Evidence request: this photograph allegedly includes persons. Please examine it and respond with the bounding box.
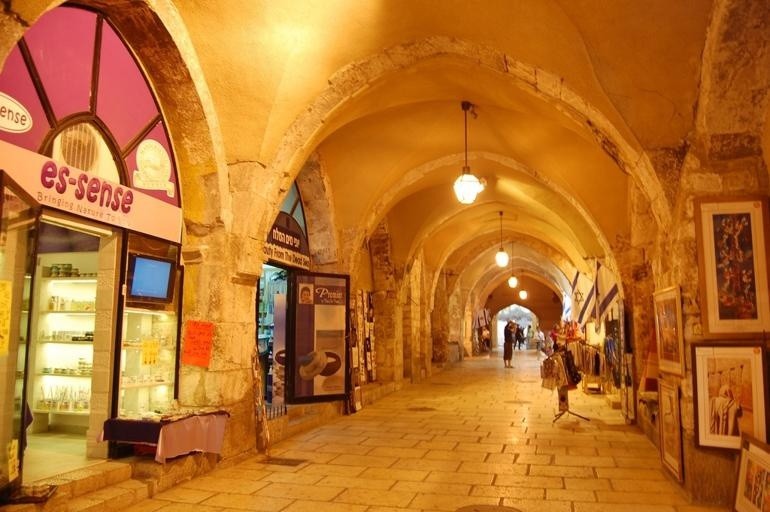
[514,323,545,351]
[504,320,516,368]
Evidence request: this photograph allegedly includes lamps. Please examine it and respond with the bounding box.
[454,100,484,206]
[492,208,528,302]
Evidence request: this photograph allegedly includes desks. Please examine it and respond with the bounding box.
[102,408,227,462]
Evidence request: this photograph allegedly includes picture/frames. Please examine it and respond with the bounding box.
[690,190,770,511]
[652,281,685,488]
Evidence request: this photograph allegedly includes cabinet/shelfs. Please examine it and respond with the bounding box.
[120,308,179,415]
[34,273,97,415]
